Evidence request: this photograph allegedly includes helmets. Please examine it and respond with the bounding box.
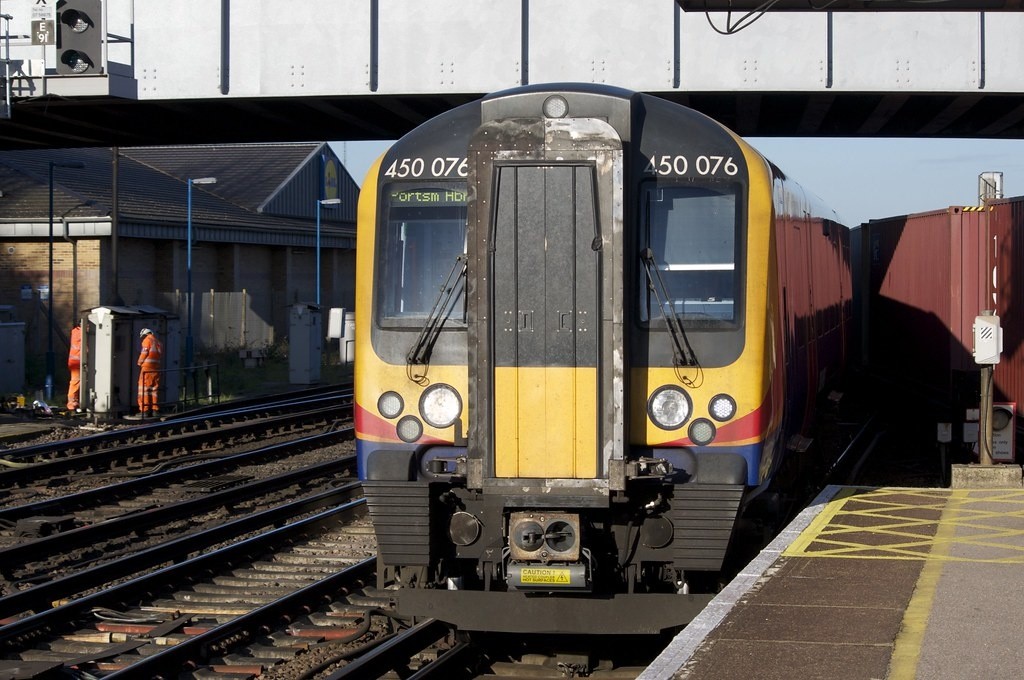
[139,328,154,339]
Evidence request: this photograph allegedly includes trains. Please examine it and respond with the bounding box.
[357,83,1023,637]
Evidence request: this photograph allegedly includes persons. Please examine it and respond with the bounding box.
[66,318,90,409]
[136,327,163,418]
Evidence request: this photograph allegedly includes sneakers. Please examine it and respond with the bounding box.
[67,403,79,411]
[135,412,149,418]
[149,409,161,417]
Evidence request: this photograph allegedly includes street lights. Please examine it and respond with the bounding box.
[184,177,216,379]
[317,199,340,307]
[44,159,82,402]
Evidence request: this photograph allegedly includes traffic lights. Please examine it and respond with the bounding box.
[54,1,106,75]
